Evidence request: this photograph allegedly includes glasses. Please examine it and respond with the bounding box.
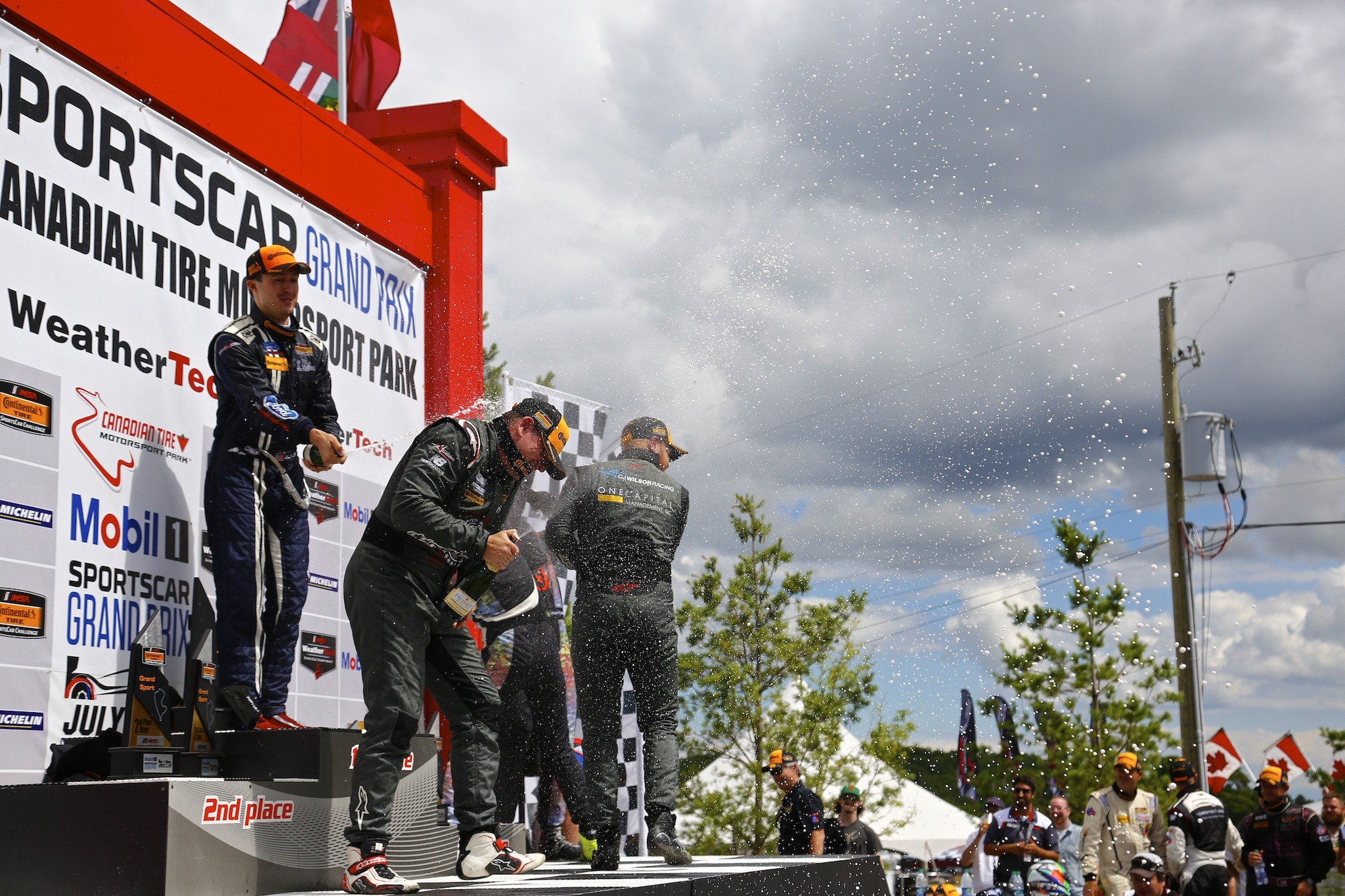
[769,768,782,775]
[1131,858,1164,872]
[843,796,858,801]
[1013,789,1030,793]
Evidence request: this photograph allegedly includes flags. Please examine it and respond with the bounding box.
[1322,750,1345,795]
[990,695,1019,793]
[1261,735,1311,783]
[1193,728,1242,794]
[1034,707,1068,797]
[957,689,977,800]
[1089,701,1113,751]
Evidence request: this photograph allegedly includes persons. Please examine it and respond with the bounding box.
[341,398,569,894]
[546,417,694,870]
[469,515,595,864]
[960,753,1345,896]
[204,246,346,731]
[826,784,884,868]
[761,749,825,856]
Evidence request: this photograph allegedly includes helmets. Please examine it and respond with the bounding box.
[1027,860,1071,896]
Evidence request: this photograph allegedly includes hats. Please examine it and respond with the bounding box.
[984,797,1006,809]
[245,245,311,278]
[840,787,860,799]
[1126,853,1166,877]
[514,398,571,481]
[1170,758,1196,782]
[762,749,798,773]
[1113,751,1141,772]
[621,417,689,462]
[1256,766,1288,787]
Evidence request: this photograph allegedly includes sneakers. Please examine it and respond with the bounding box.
[341,837,421,895]
[268,712,304,727]
[538,826,582,861]
[456,823,545,880]
[590,830,621,871]
[579,825,597,839]
[254,716,280,729]
[644,814,692,866]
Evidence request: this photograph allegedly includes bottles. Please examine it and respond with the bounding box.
[961,869,974,896]
[916,868,927,896]
[1254,850,1268,885]
[440,535,519,623]
[1023,840,1032,862]
[302,443,341,469]
[1009,871,1024,896]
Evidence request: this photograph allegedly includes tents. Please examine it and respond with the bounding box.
[675,679,986,896]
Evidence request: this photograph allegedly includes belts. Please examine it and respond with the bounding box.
[1268,875,1306,887]
